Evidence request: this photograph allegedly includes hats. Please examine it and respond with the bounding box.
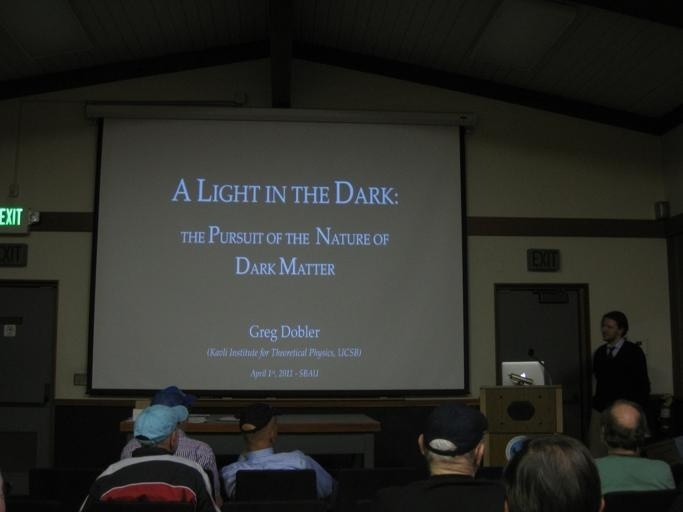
[134,403,189,445]
[238,402,278,434]
[422,402,489,457]
[150,386,200,408]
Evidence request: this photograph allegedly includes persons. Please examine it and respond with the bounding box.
[91,386,334,512]
[374,400,675,512]
[591,312,648,415]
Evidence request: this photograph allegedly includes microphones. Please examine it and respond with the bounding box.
[527,348,553,386]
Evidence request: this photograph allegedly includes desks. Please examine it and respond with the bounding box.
[119,413,381,472]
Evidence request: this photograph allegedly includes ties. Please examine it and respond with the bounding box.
[607,346,616,358]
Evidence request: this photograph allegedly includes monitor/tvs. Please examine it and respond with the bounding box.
[501,360,545,387]
